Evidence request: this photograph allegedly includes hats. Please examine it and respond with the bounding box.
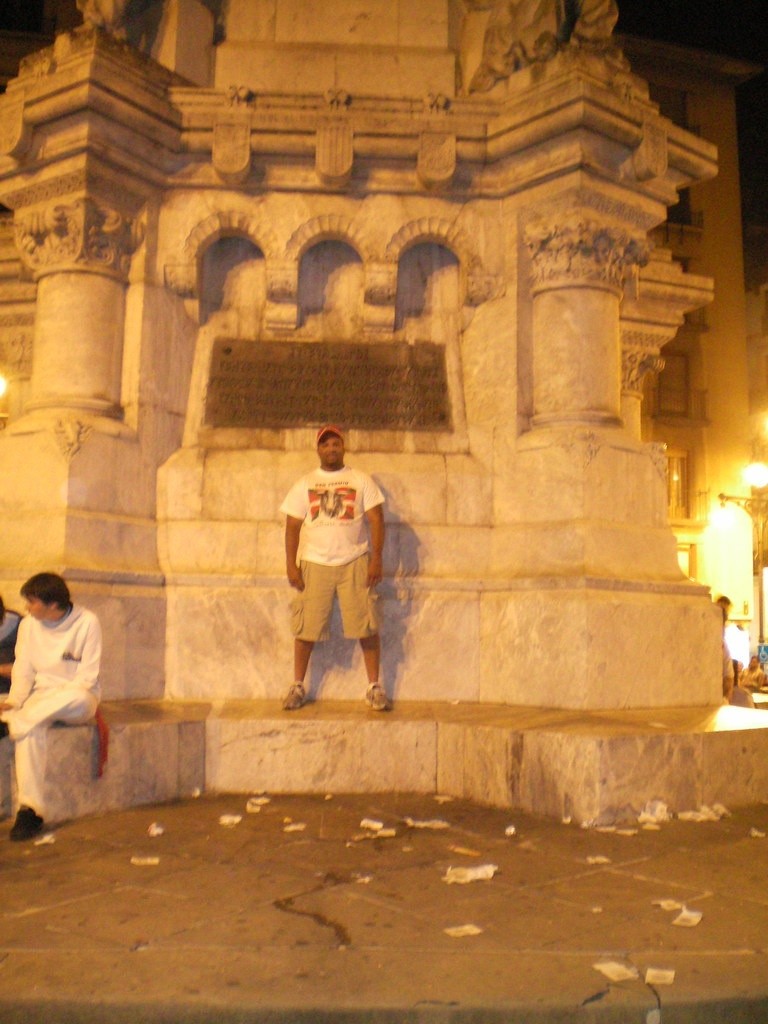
[317,424,344,445]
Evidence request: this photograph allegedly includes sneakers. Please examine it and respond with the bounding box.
[282,685,307,709]
[366,686,392,709]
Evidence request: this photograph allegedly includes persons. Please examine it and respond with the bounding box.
[715,596,768,709]
[279,423,392,712]
[0,572,102,840]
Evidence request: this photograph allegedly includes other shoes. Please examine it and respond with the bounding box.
[10,806,44,840]
